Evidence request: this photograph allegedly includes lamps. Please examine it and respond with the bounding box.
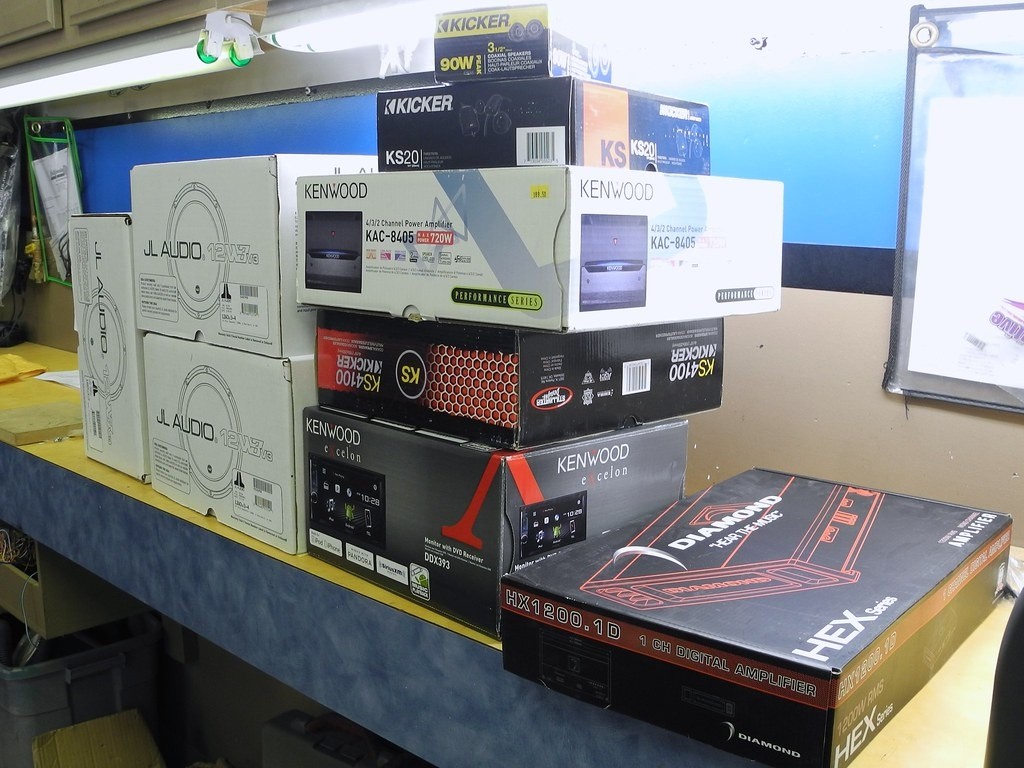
[0,9,258,110]
[257,0,432,54]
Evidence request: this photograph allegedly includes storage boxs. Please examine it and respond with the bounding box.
[129,153,376,358]
[0,612,162,768]
[304,404,690,641]
[377,78,710,174]
[143,331,314,554]
[499,468,1013,768]
[434,4,612,83]
[314,308,725,451]
[67,212,152,484]
[296,166,783,334]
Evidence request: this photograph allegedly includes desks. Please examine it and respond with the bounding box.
[0,343,1024,768]
